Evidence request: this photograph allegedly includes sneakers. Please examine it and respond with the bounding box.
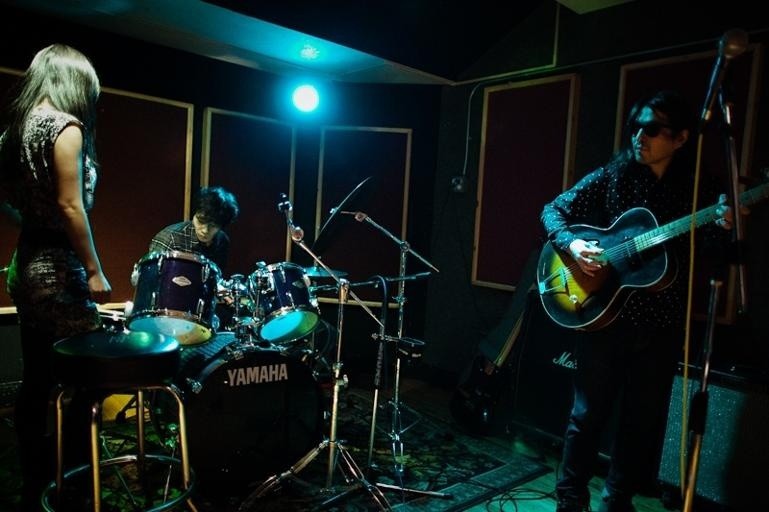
[555,492,594,512]
[596,494,638,512]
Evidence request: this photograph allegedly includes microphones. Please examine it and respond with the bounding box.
[285,209,303,243]
[700,27,748,127]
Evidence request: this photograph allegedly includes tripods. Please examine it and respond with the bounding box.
[238,280,392,512]
[319,303,453,508]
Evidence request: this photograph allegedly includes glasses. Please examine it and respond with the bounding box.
[628,119,675,138]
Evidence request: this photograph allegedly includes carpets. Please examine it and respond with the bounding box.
[0,381,554,512]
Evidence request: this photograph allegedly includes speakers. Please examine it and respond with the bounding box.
[656,361,769,511]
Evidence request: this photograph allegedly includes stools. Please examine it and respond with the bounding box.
[42,331,200,512]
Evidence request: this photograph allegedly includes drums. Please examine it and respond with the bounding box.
[125,250,221,346]
[150,332,327,512]
[245,261,322,345]
[281,346,329,379]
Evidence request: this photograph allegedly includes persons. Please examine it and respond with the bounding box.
[539,83,750,512]
[147,186,240,272]
[0,44,112,512]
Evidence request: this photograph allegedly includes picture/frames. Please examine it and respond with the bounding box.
[314,128,412,311]
[470,70,581,297]
[1,67,25,316]
[200,106,297,281]
[85,88,196,306]
[613,40,761,326]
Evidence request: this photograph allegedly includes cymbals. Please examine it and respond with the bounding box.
[302,265,352,280]
[53,327,179,358]
[310,175,374,257]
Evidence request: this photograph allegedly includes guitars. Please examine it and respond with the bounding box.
[537,182,769,332]
